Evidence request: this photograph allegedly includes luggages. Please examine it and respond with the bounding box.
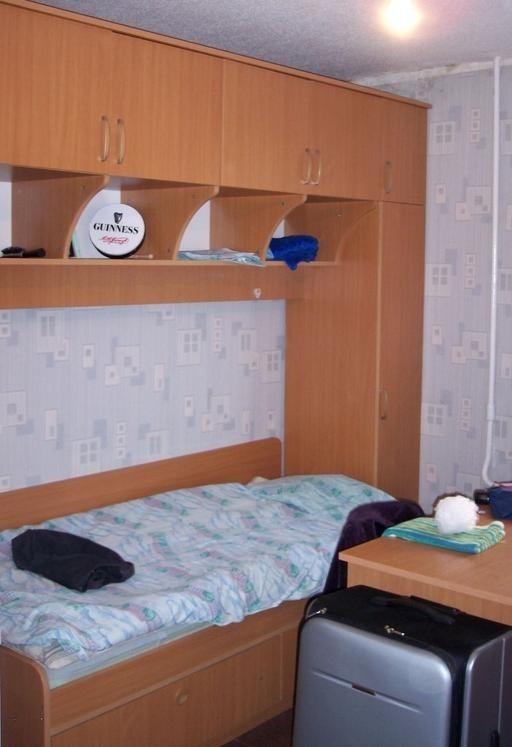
[293,585,512,746]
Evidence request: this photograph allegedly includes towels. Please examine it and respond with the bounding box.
[383,515,506,554]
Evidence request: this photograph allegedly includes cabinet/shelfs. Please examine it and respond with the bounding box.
[0,162,366,311]
[371,86,432,204]
[216,51,379,212]
[0,0,217,194]
[282,195,425,510]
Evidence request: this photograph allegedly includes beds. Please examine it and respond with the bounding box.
[1,430,407,747]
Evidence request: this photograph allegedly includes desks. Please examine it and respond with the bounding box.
[338,483,512,621]
